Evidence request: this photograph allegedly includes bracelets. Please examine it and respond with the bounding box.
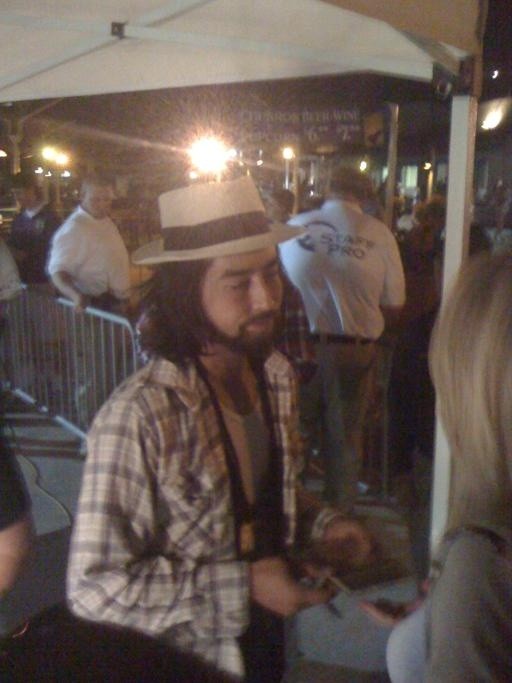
[307,504,339,557]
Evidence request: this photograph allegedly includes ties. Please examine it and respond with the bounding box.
[221,359,282,561]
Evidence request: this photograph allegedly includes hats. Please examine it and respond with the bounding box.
[129,176,309,269]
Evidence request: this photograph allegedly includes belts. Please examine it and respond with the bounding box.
[309,331,375,347]
[90,298,129,308]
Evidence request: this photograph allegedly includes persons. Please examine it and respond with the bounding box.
[0,426,41,606]
[8,170,62,291]
[353,247,511,682]
[267,167,493,522]
[48,170,132,317]
[60,171,387,679]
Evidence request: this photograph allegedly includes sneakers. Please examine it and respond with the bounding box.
[1,604,30,641]
